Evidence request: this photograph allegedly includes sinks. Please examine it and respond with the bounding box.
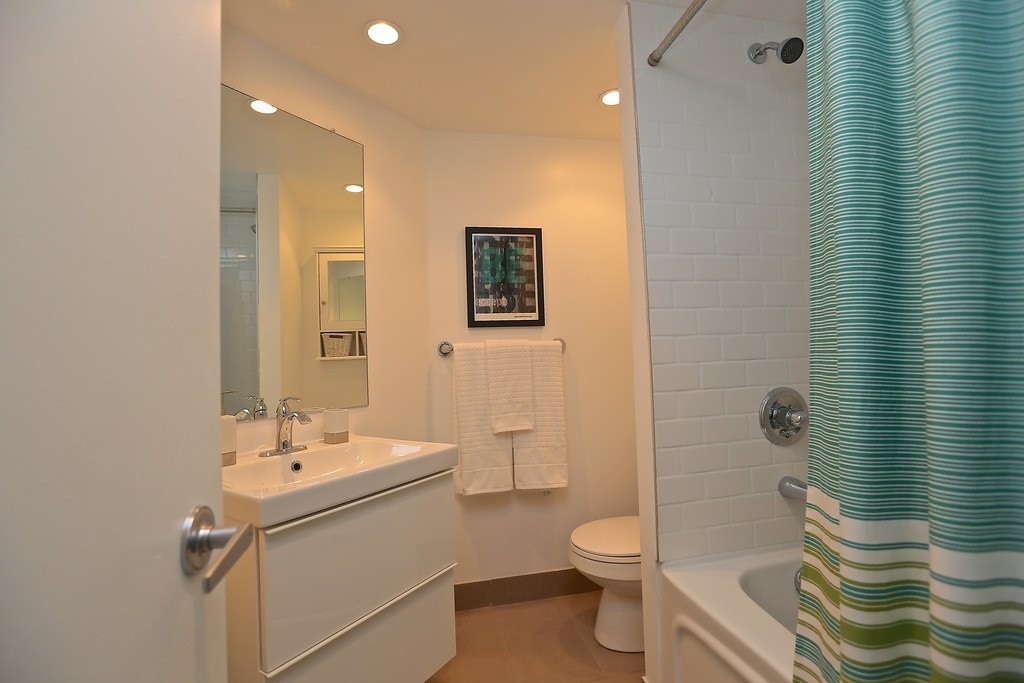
[222,441,421,488]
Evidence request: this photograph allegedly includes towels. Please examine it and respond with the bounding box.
[453,341,568,496]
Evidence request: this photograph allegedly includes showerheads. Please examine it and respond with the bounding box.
[776,36,805,65]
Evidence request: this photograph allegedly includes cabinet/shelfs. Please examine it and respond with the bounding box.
[223,467,462,682]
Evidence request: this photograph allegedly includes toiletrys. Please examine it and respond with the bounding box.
[221,389,238,467]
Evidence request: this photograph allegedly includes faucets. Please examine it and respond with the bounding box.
[258,397,312,457]
[779,476,806,502]
[234,395,268,420]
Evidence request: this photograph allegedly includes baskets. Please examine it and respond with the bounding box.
[321,333,352,356]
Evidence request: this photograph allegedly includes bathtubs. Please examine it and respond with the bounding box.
[658,541,804,683]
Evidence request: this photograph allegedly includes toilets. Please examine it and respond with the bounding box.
[568,516,644,652]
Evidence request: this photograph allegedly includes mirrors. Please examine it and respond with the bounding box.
[220,81,369,422]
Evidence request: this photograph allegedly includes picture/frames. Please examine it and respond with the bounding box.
[465,226,546,328]
